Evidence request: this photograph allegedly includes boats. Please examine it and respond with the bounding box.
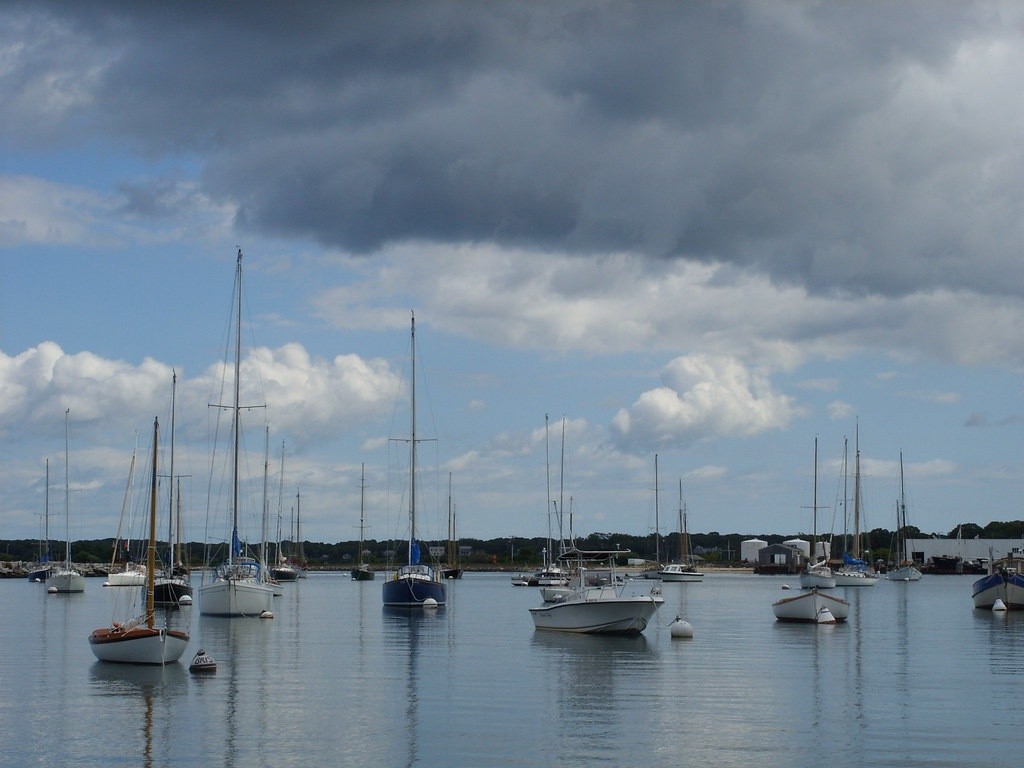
[528,540,666,636]
[971,562,1024,610]
[771,587,851,625]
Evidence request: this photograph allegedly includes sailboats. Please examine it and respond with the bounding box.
[103,364,195,606]
[885,447,923,582]
[797,435,838,589]
[511,410,705,604]
[46,406,86,593]
[27,457,61,584]
[827,413,880,587]
[380,307,449,607]
[217,423,308,583]
[88,416,191,666]
[436,471,464,580]
[349,460,376,581]
[195,244,275,619]
